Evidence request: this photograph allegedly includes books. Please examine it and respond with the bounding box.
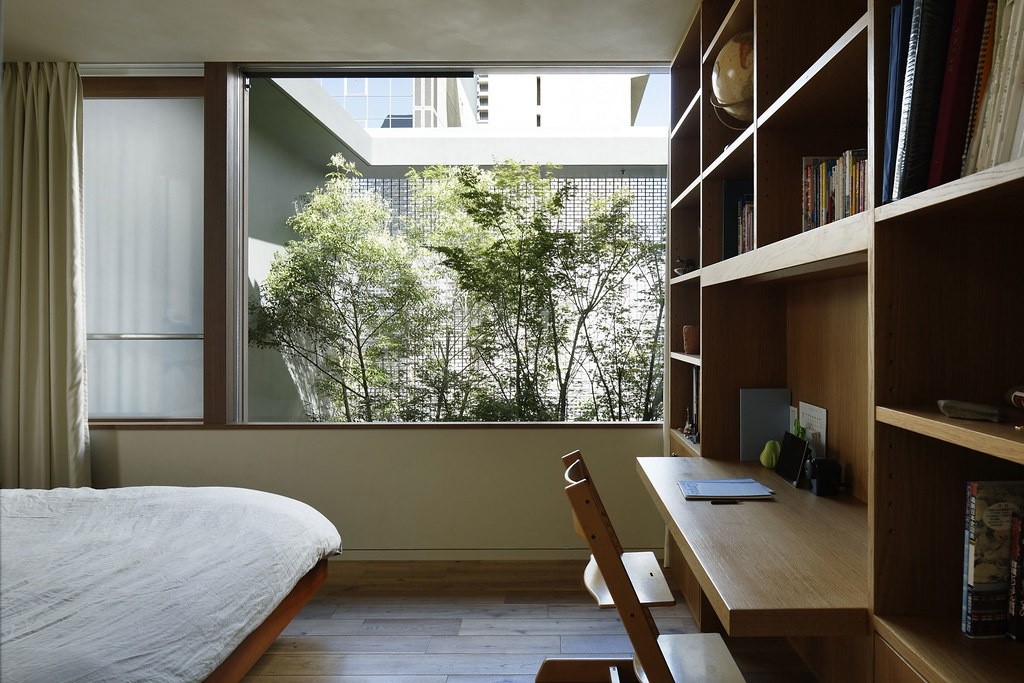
[963,480,1024,643]
[881,0,1024,205]
[737,200,754,255]
[802,149,868,232]
[677,477,776,500]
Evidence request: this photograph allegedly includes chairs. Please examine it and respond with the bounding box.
[535,451,749,683]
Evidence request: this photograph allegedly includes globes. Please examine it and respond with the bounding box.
[708,27,754,130]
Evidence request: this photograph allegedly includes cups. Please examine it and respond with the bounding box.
[684,324,700,355]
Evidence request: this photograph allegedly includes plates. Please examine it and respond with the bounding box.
[673,268,692,275]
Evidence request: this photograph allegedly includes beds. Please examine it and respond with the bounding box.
[0,486,343,683]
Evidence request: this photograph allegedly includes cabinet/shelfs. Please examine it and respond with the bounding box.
[634,0,1024,683]
